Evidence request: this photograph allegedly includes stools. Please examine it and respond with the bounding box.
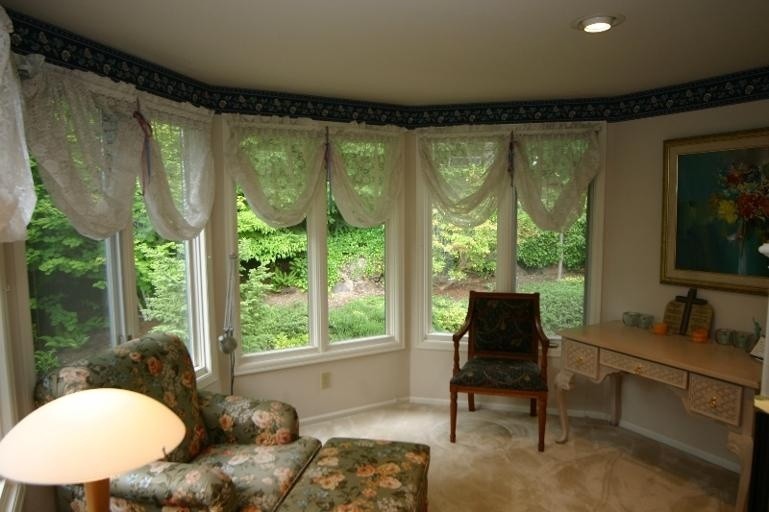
[273,436,430,512]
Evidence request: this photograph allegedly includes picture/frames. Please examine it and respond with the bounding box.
[659,127,769,296]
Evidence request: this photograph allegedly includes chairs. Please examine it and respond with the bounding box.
[449,290,551,453]
[31,329,322,512]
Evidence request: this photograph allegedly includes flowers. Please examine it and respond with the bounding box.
[708,159,769,258]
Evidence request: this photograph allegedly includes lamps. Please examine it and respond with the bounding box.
[0,388,187,512]
[581,16,615,34]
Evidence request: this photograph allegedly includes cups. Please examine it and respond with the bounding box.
[716,328,754,349]
[623,312,653,330]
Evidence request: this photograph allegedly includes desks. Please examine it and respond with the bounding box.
[553,318,764,512]
